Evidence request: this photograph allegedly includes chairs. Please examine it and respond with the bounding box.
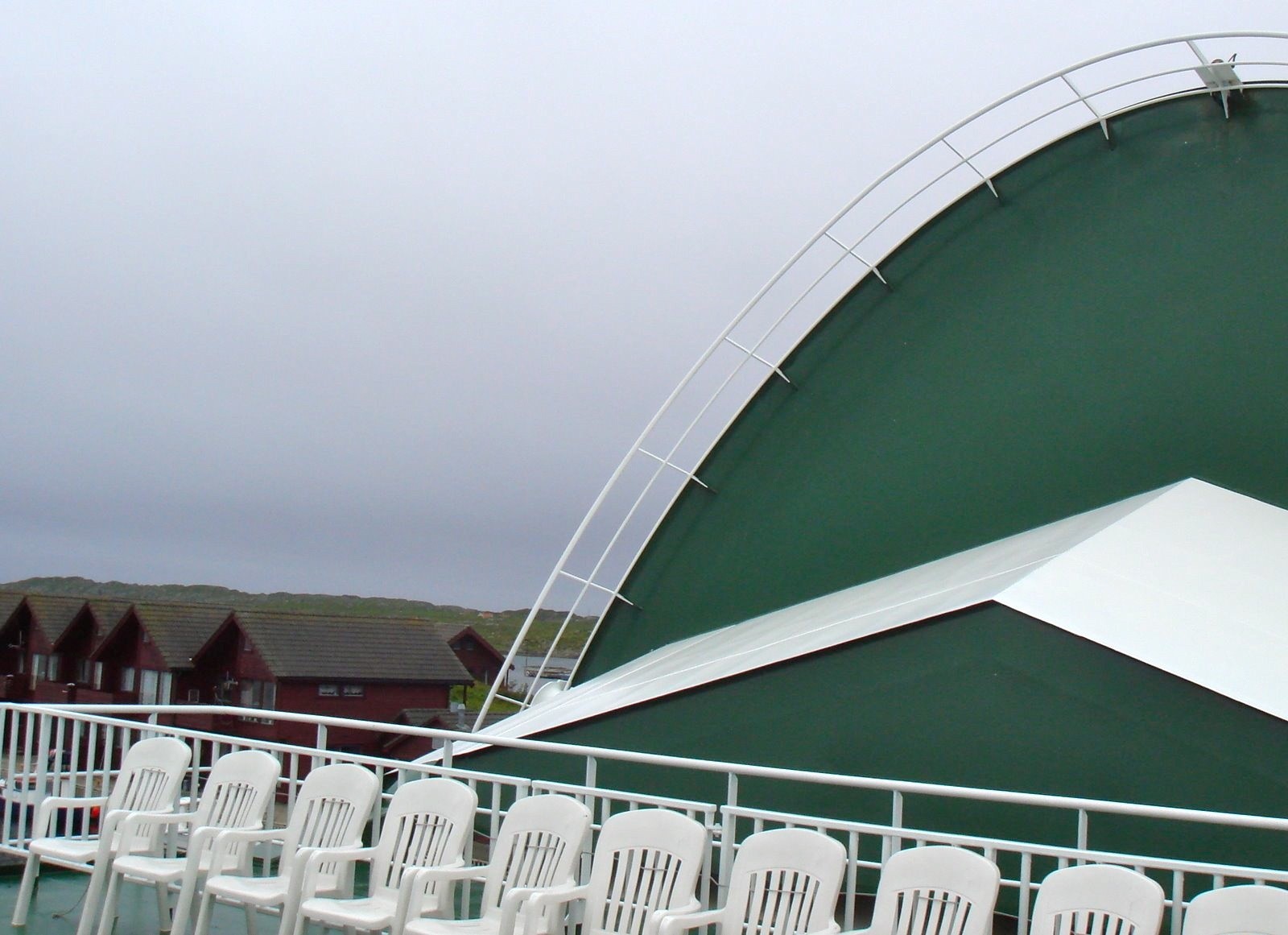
[96,750,281,935]
[658,826,843,935]
[524,809,705,935]
[1028,864,1165,935]
[1182,885,1288,935]
[839,844,1001,935]
[403,794,590,935]
[12,736,192,935]
[292,778,479,935]
[195,764,379,935]
